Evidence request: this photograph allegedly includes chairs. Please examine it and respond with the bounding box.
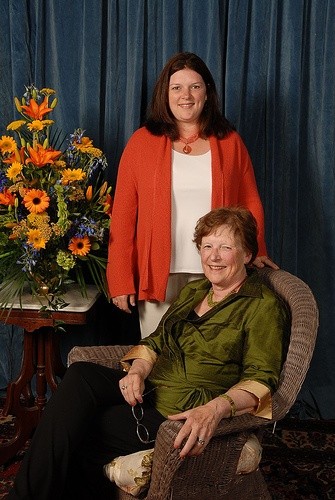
[66,269,319,500]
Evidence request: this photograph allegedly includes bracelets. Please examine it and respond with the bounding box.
[219,394,236,418]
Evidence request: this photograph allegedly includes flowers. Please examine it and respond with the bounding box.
[0,85,115,340]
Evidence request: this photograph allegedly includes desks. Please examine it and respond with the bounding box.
[0,282,103,472]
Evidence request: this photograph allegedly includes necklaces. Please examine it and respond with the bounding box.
[175,128,204,154]
[207,276,247,308]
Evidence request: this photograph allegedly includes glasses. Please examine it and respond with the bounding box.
[131,386,158,445]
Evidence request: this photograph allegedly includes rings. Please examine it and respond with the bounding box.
[198,440,206,445]
[121,386,128,390]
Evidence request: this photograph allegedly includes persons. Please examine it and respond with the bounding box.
[108,52,279,341]
[13,207,290,500]
